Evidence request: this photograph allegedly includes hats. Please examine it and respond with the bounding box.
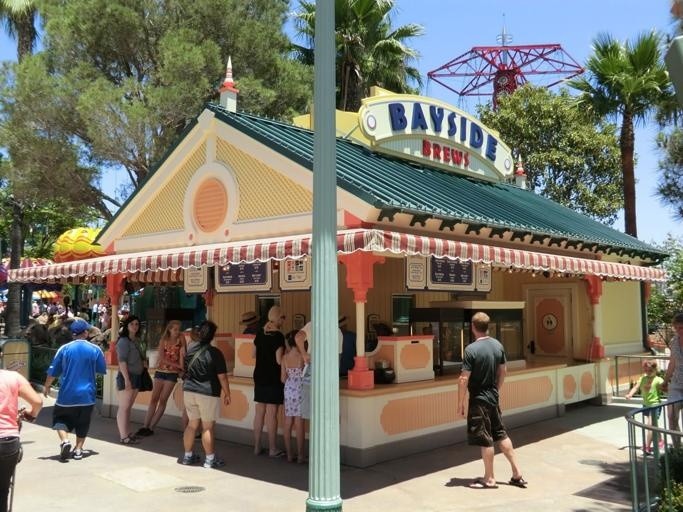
[338,315,349,326]
[71,320,91,336]
[239,312,258,325]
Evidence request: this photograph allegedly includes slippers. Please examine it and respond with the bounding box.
[270,449,285,457]
[469,480,498,488]
[509,477,527,485]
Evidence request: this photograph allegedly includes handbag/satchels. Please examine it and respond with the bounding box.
[138,367,153,392]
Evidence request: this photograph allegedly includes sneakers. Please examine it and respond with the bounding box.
[60,441,71,462]
[204,457,224,467]
[135,428,153,436]
[643,446,652,455]
[658,440,664,449]
[73,447,83,459]
[183,455,200,465]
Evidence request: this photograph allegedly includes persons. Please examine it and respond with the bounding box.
[134,320,187,437]
[624,354,668,453]
[661,313,682,442]
[0,288,130,341]
[253,306,356,463]
[44,319,106,459]
[115,314,149,443]
[183,320,231,467]
[457,313,527,491]
[0,368,44,509]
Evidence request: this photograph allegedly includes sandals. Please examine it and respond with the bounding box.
[119,432,143,446]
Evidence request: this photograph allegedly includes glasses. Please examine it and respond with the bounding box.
[281,315,286,320]
